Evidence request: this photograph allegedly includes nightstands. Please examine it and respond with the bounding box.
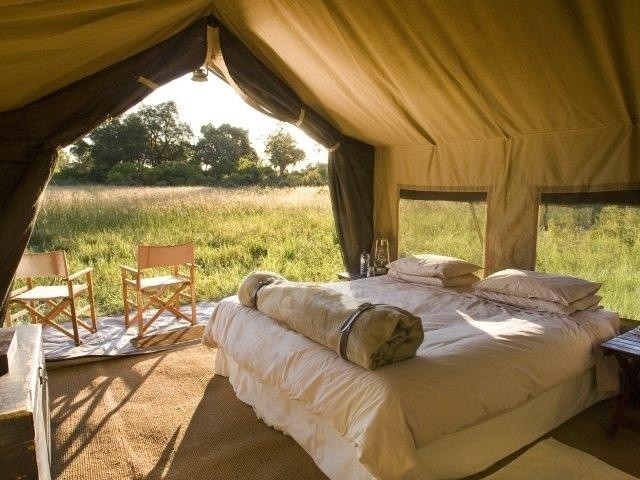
[599,324,640,440]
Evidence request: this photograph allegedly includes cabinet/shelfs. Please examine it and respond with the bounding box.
[1,323,53,480]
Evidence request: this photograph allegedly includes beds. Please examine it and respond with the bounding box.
[215,275,620,479]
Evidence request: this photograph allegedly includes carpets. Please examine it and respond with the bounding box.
[479,436,639,480]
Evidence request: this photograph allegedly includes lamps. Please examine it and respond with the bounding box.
[190,62,209,82]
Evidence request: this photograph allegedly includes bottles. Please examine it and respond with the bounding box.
[360,249,370,278]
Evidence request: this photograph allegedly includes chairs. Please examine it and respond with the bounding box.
[4,240,197,347]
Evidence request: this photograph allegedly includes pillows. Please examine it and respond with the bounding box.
[384,253,604,317]
